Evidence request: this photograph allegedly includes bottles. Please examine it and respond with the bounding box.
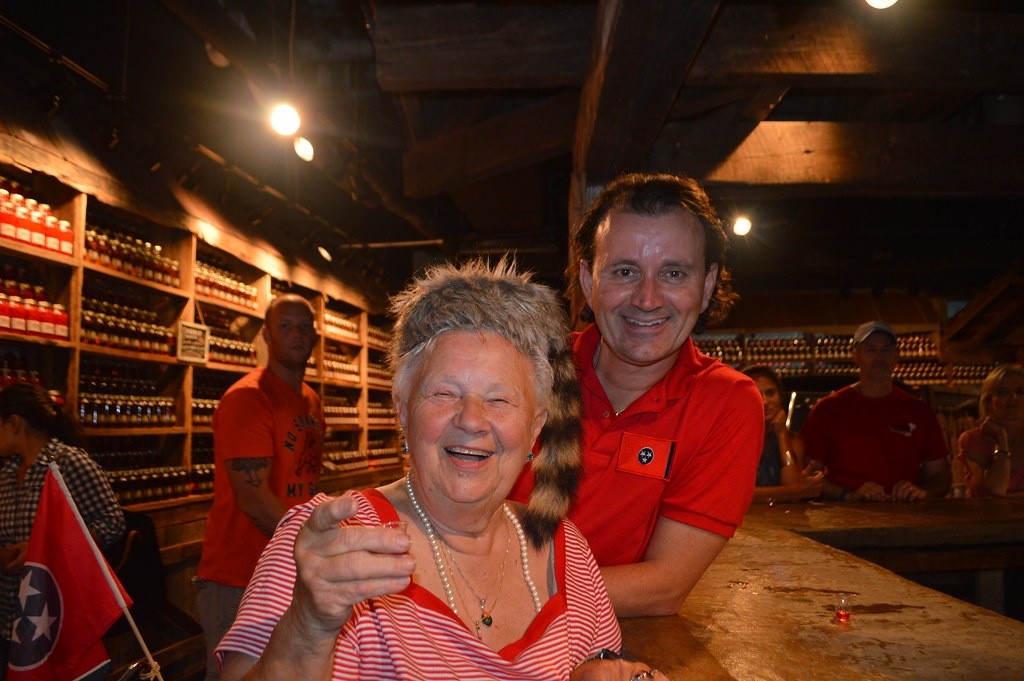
[896,335,938,362]
[367,401,396,427]
[192,381,224,426]
[196,312,256,367]
[694,337,742,373]
[953,362,994,385]
[367,324,390,349]
[325,308,359,341]
[745,336,812,376]
[323,395,359,425]
[814,336,860,376]
[935,406,974,451]
[320,441,399,473]
[0,176,179,427]
[323,347,360,383]
[195,260,289,310]
[367,361,392,387]
[892,362,948,386]
[91,447,215,506]
[304,357,317,377]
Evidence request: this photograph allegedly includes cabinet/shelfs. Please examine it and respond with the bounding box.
[691,323,1023,423]
[0,130,401,613]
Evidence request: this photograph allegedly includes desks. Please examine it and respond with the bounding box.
[609,496,1024,680]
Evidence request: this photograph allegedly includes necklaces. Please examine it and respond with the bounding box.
[593,343,600,370]
[406,471,541,639]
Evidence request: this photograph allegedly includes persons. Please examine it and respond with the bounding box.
[798,321,952,503]
[507,174,766,618]
[208,262,667,681]
[954,365,1024,501]
[739,364,824,505]
[0,378,122,640]
[196,295,327,681]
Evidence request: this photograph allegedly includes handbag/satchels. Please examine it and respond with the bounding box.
[104,505,165,638]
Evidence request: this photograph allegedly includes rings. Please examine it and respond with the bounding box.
[630,668,658,681]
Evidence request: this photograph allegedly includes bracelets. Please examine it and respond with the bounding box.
[993,449,1010,457]
[782,450,797,466]
[584,649,624,661]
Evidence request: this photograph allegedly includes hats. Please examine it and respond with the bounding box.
[853,321,897,348]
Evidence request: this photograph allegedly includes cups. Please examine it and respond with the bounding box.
[376,522,409,556]
[834,594,854,620]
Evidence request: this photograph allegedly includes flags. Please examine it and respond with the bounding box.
[6,469,134,681]
